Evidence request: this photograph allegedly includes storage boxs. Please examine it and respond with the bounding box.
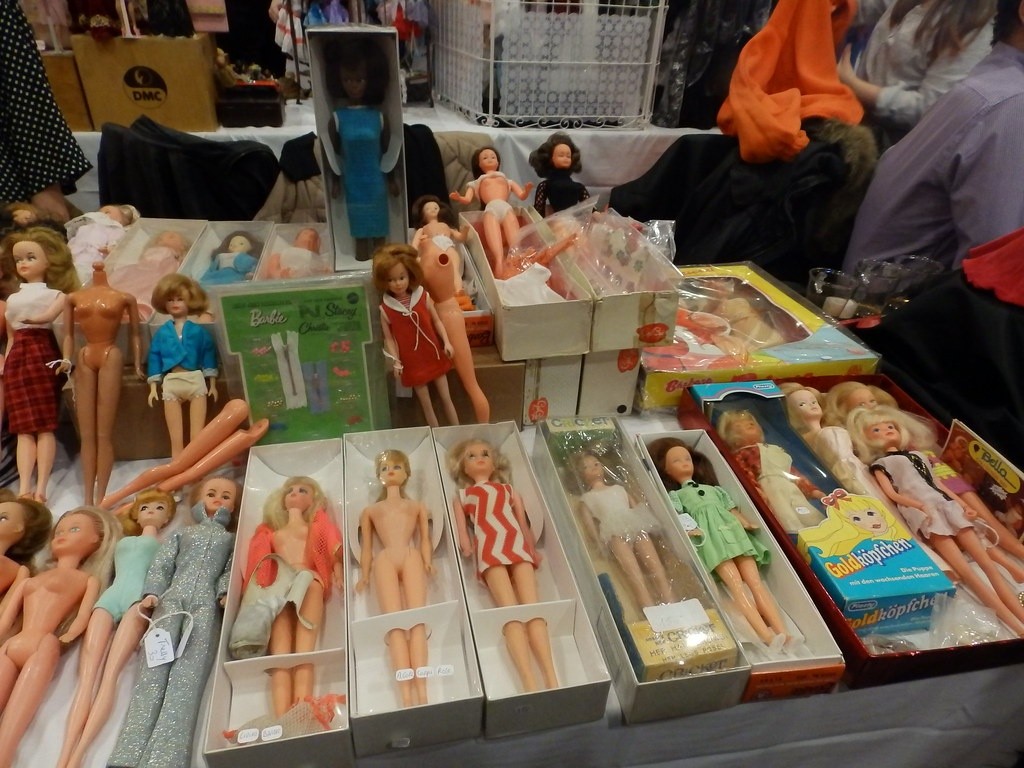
[306,24,408,270]
[255,222,334,290]
[636,430,846,701]
[677,372,1024,693]
[634,266,878,419]
[39,49,92,131]
[524,358,584,427]
[342,427,485,759]
[408,228,495,347]
[150,219,274,367]
[460,207,594,361]
[528,204,679,353]
[689,376,953,636]
[430,419,613,744]
[70,32,217,130]
[202,438,356,768]
[532,416,752,728]
[53,216,208,369]
[578,348,641,419]
[62,338,524,461]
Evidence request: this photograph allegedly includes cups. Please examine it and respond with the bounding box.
[854,261,903,316]
[890,254,945,309]
[807,268,857,319]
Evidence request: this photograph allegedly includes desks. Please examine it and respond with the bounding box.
[0,438,1024,768]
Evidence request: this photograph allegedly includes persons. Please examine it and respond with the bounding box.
[0,51,1024,768]
[833,1,1024,308]
[0,1,93,221]
